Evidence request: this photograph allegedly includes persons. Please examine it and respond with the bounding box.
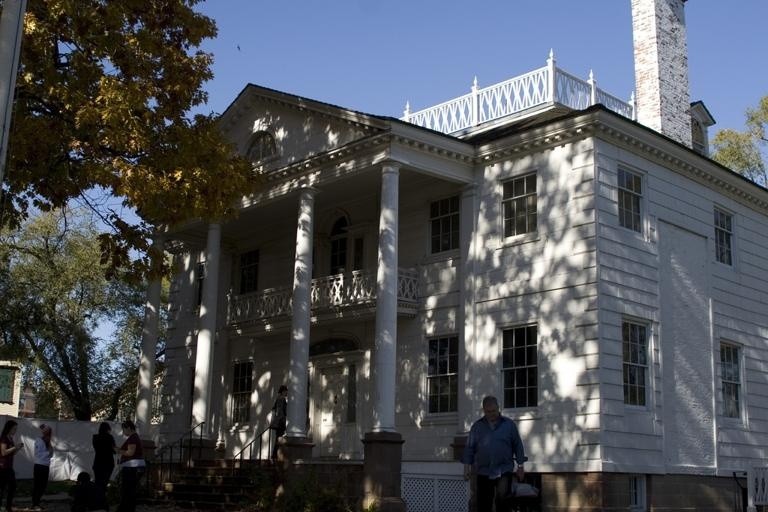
[88,422,116,491]
[114,421,146,483]
[0,419,24,511]
[31,423,53,507]
[460,396,528,511]
[270,385,288,459]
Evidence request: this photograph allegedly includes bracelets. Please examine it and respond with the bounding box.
[518,464,523,467]
